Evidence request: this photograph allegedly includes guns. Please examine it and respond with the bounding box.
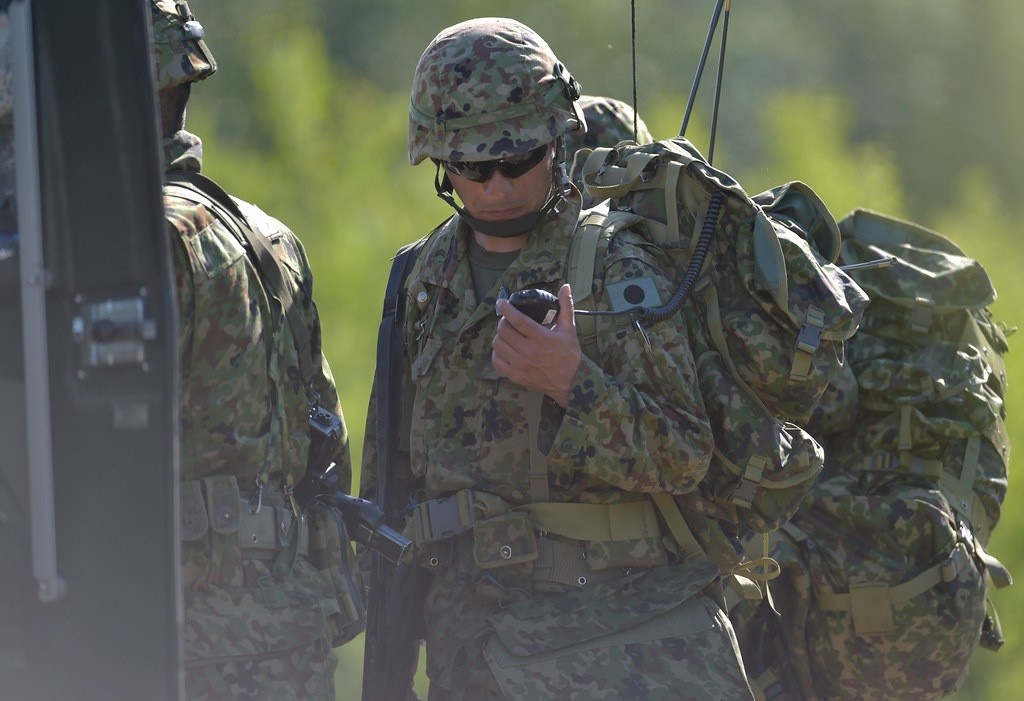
[291,405,413,565]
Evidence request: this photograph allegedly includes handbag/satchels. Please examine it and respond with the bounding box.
[301,499,370,647]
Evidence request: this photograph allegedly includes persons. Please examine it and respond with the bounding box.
[359,17,758,701]
[149,0,366,701]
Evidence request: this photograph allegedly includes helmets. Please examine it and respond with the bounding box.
[565,94,653,182]
[407,17,588,237]
[149,0,218,90]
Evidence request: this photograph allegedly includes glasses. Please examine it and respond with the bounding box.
[441,143,549,183]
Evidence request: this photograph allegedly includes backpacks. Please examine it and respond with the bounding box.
[404,136,871,619]
[752,207,1014,701]
